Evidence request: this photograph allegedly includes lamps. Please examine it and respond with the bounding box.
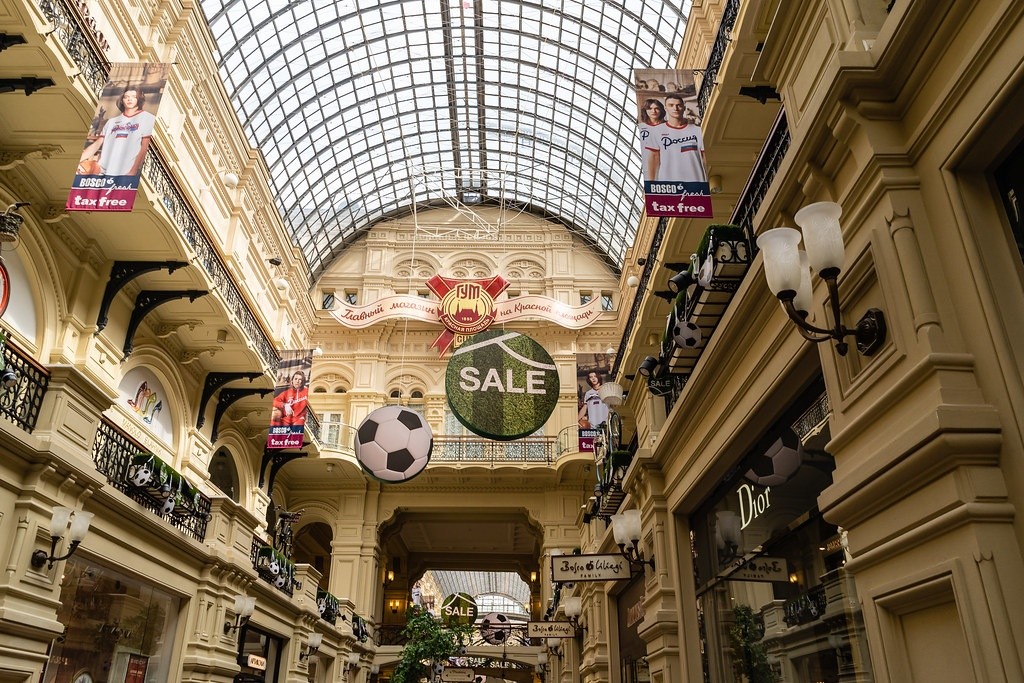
[343,653,360,676]
[31,507,95,570]
[224,595,256,634]
[300,633,323,660]
[547,638,564,660]
[610,510,656,573]
[639,356,658,377]
[668,270,693,294]
[383,571,394,587]
[390,600,399,613]
[756,201,887,357]
[565,597,588,633]
[537,654,551,675]
[594,484,603,497]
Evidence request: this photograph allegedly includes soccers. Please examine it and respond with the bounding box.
[268,563,285,587]
[673,253,713,351]
[134,468,175,513]
[354,405,433,483]
[480,614,511,643]
[317,599,326,613]
[740,428,802,485]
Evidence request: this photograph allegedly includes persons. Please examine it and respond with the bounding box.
[270,371,308,425]
[638,95,709,181]
[578,371,610,429]
[80,87,156,175]
